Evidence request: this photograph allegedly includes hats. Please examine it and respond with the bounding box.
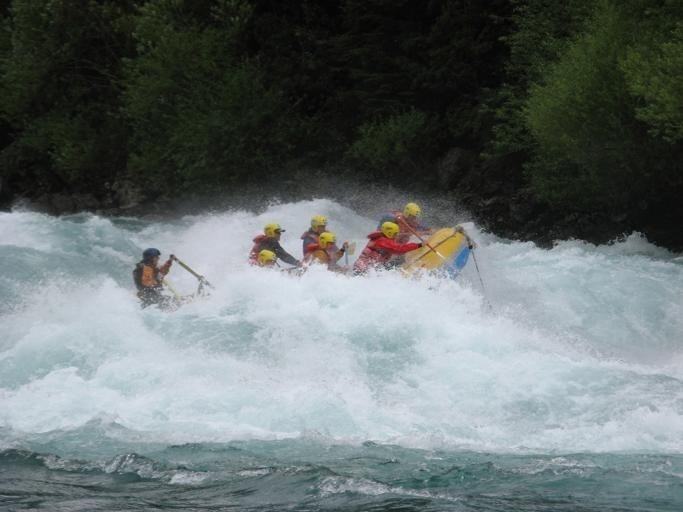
[275,229,286,233]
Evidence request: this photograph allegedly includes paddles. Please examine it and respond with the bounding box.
[346,241,357,256]
[399,216,464,278]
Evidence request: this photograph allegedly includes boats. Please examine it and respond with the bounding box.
[395,224,473,288]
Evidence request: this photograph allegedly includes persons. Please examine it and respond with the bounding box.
[133,247,174,311]
[247,202,435,276]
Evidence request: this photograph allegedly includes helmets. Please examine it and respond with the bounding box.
[319,232,336,248]
[258,249,276,263]
[264,223,280,237]
[311,214,326,232]
[381,221,399,239]
[143,248,160,258]
[403,203,421,218]
